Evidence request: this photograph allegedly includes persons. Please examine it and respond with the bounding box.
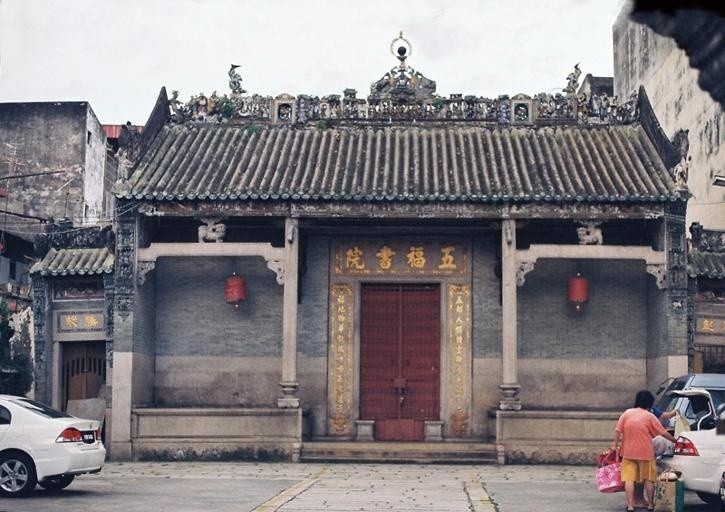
[630,402,680,509]
[610,388,678,512]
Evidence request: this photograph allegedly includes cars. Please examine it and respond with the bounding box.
[0,394,105,498]
[656,373,725,505]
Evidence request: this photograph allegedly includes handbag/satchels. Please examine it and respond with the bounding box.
[596,448,624,495]
[653,466,686,512]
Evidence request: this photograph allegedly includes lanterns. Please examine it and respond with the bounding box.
[223,272,248,313]
[566,270,591,315]
